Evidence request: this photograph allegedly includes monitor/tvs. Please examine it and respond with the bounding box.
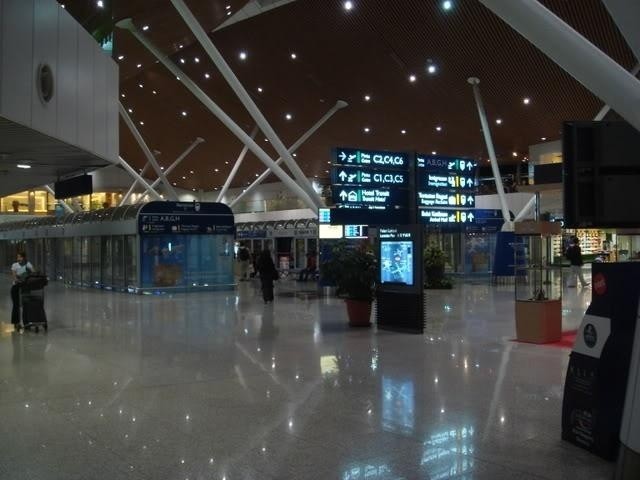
[318,207,331,223]
[318,223,344,239]
[345,223,369,240]
[563,120,640,229]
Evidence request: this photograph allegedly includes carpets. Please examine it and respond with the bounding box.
[511,329,577,348]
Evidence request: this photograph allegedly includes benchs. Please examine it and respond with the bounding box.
[276,267,320,280]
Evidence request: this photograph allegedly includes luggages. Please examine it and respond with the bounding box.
[22,295,47,325]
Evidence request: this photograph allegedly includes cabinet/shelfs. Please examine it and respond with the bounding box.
[511,220,563,341]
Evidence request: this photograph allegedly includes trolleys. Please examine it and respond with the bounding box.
[15,271,48,333]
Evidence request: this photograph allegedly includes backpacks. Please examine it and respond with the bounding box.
[238,247,250,261]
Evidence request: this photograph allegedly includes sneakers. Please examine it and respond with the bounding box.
[263,300,273,302]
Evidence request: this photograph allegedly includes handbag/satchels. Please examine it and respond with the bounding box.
[257,263,278,279]
[21,271,45,291]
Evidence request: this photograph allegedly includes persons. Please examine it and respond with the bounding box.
[255,249,275,306]
[236,241,252,281]
[390,251,405,282]
[297,252,317,282]
[250,249,258,275]
[564,237,591,289]
[566,235,576,247]
[9,250,36,332]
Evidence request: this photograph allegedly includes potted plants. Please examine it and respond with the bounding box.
[422,241,445,279]
[335,250,384,326]
